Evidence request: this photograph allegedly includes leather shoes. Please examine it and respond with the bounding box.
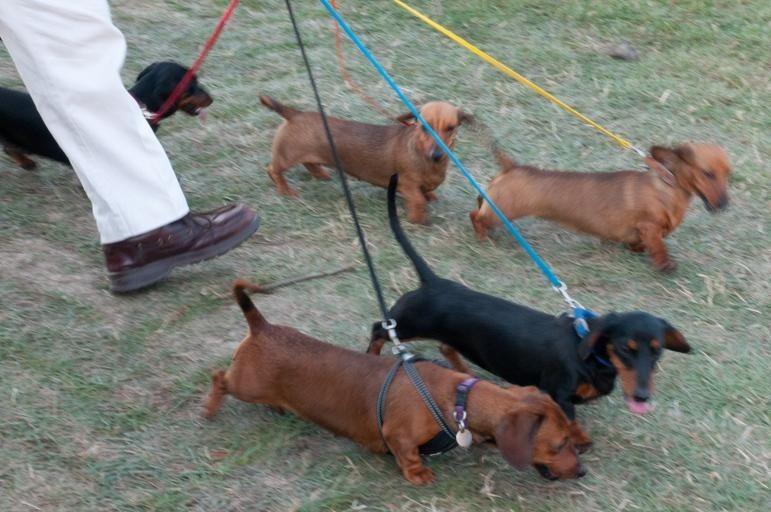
[103,205,257,292]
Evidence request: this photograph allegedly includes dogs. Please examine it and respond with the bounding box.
[364,171,696,457]
[198,279,589,488]
[0,61,214,172]
[258,92,473,226]
[468,123,733,274]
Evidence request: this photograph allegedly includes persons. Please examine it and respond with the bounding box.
[0,1,260,295]
